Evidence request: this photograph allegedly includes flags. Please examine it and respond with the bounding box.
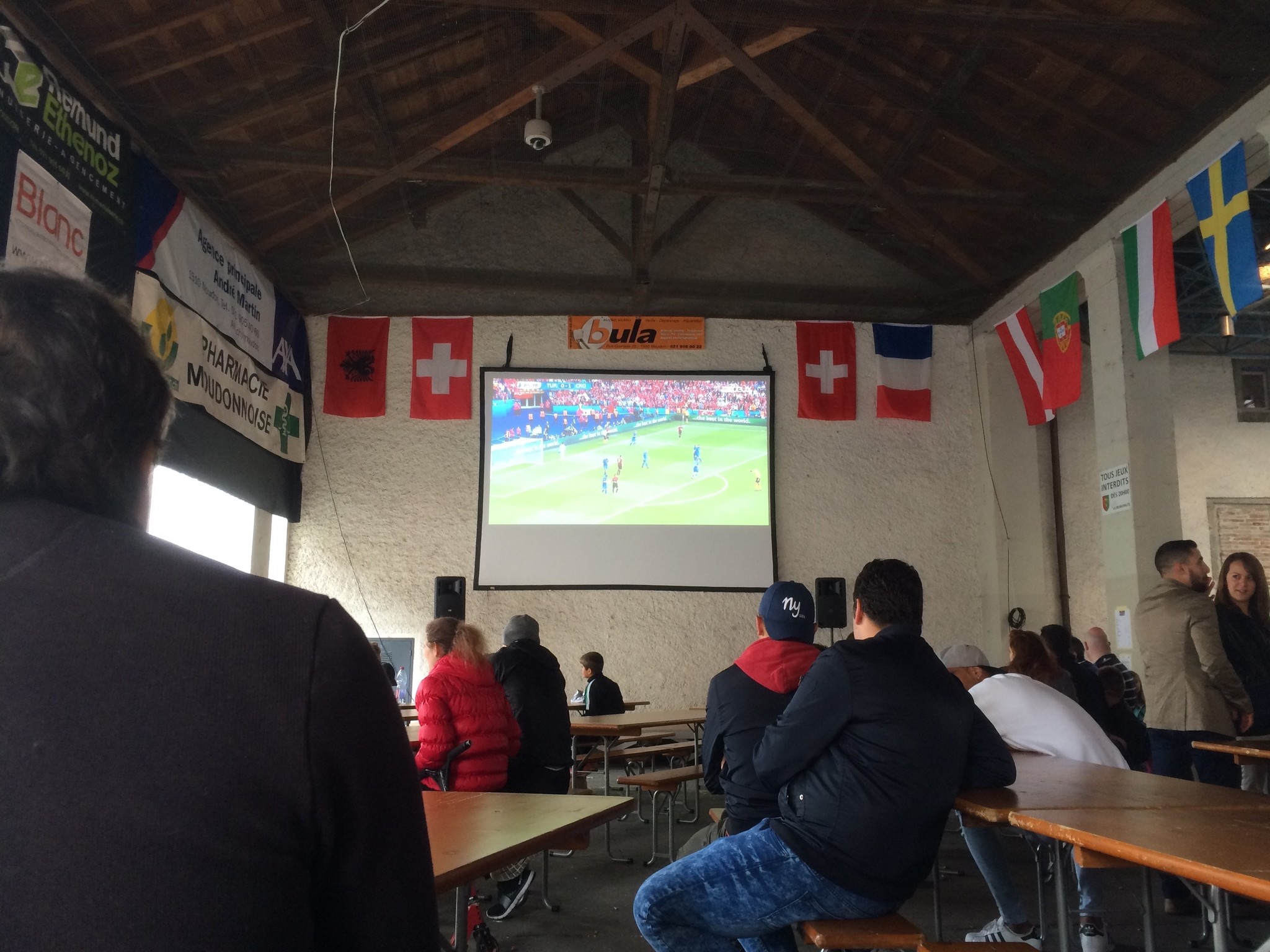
[0,15,472,524]
[796,140,1266,426]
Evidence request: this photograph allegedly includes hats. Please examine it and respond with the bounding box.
[941,644,1004,674]
[759,580,815,644]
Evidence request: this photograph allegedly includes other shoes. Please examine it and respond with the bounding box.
[1164,895,1198,915]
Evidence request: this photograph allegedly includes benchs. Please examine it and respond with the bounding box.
[572,732,707,869]
[709,806,927,951]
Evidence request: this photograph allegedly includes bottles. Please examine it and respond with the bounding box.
[395,666,407,705]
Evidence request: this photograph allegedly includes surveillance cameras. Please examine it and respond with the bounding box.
[524,119,553,150]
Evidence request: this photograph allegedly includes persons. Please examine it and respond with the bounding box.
[938,624,1147,952]
[483,613,571,919]
[0,267,443,952]
[570,652,625,777]
[632,559,1017,952]
[415,616,520,952]
[371,641,397,693]
[492,378,769,499]
[1215,553,1270,798]
[1134,538,1253,915]
[675,579,829,861]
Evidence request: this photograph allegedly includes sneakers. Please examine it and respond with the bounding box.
[1079,923,1116,952]
[965,916,1042,951]
[486,868,535,920]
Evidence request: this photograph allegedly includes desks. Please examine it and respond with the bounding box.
[1191,741,1270,765]
[933,752,1270,952]
[421,789,634,952]
[550,709,704,864]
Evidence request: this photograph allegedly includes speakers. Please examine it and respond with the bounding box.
[815,577,847,628]
[433,576,466,621]
